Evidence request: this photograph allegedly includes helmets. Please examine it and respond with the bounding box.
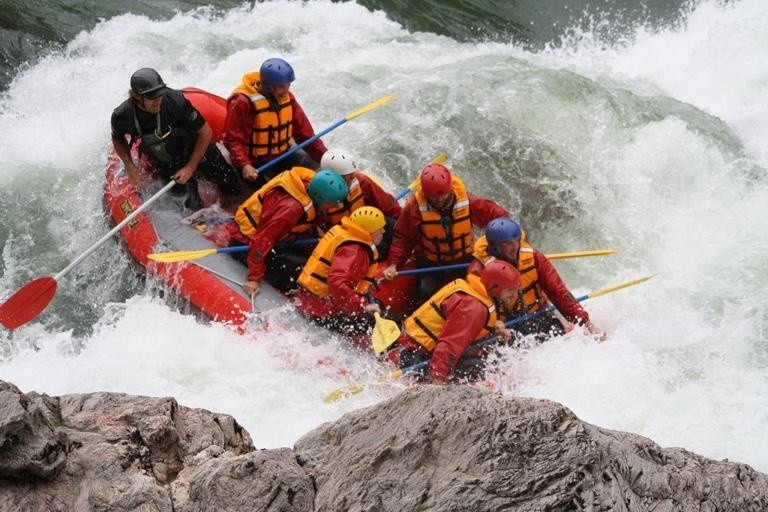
[308,169,347,202]
[260,58,295,84]
[129,67,166,94]
[420,163,451,195]
[485,218,520,245]
[480,260,520,290]
[350,206,385,233]
[319,147,358,174]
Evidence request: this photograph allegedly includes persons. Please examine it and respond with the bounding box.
[381,161,512,302]
[110,67,243,213]
[221,57,330,193]
[290,205,407,338]
[396,258,523,386]
[465,215,608,351]
[316,148,404,260]
[226,165,351,298]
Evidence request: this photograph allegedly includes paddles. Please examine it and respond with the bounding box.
[387,249,616,276]
[323,330,515,404]
[148,240,321,264]
[395,151,445,200]
[370,290,402,358]
[495,274,661,330]
[0,175,183,329]
[255,92,400,174]
